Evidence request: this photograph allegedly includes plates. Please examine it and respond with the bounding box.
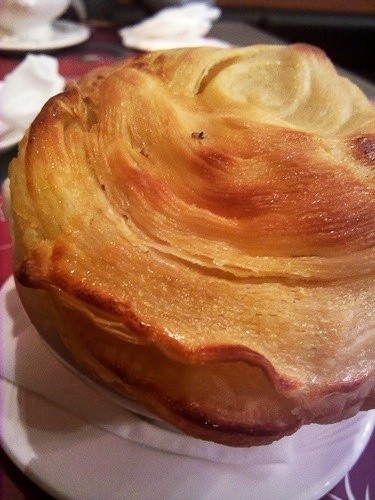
[0,112,24,155]
[0,272,374,500]
[0,18,92,52]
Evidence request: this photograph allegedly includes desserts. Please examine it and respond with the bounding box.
[8,44,375,450]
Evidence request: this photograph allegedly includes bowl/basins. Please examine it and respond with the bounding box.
[0,0,72,41]
